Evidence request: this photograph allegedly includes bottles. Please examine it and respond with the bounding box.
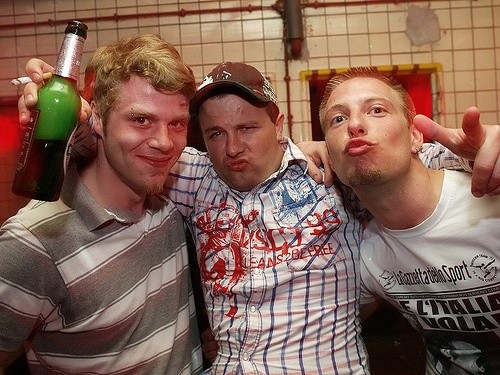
[12,19,88,202]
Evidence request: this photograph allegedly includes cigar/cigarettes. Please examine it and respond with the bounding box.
[6,71,53,88]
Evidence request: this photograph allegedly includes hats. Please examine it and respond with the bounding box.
[189,61,280,117]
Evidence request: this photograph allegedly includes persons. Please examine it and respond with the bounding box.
[1,29,334,375]
[201,65,499,375]
[16,57,499,375]
